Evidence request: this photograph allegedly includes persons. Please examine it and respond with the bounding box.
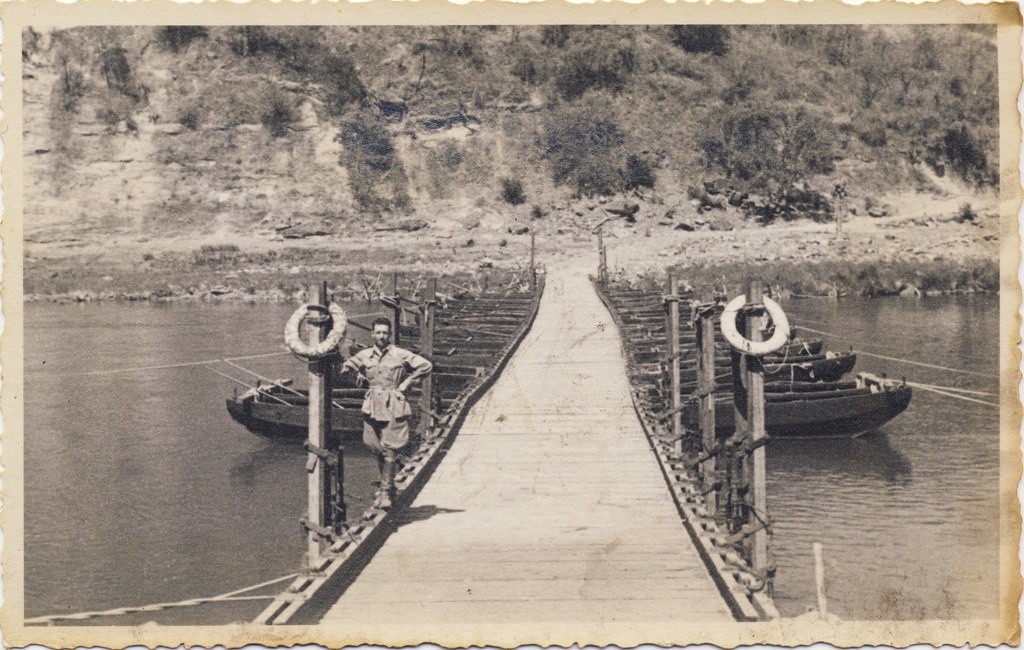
[340,317,433,508]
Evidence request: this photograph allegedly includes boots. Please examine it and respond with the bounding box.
[381,462,395,508]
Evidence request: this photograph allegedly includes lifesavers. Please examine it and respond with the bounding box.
[721,295,790,354]
[283,302,347,359]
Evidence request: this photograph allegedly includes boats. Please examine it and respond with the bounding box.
[598,280,913,438]
[228,283,543,445]
